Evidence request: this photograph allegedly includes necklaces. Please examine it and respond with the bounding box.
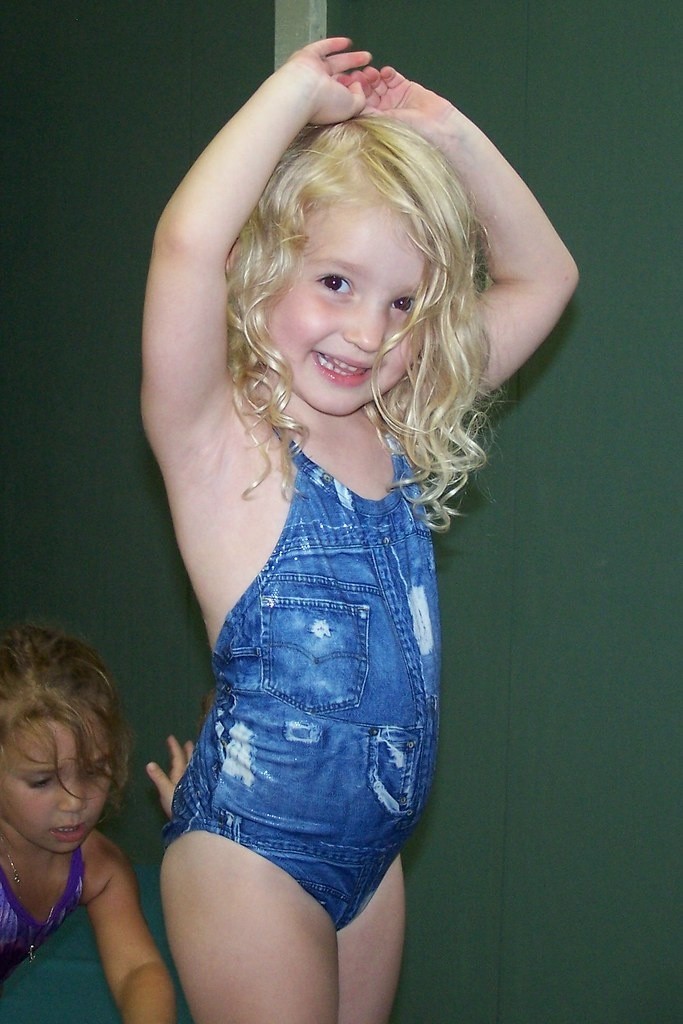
[0,833,66,964]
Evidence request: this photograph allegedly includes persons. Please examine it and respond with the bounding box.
[0,628,217,1024]
[141,37,579,1024]
